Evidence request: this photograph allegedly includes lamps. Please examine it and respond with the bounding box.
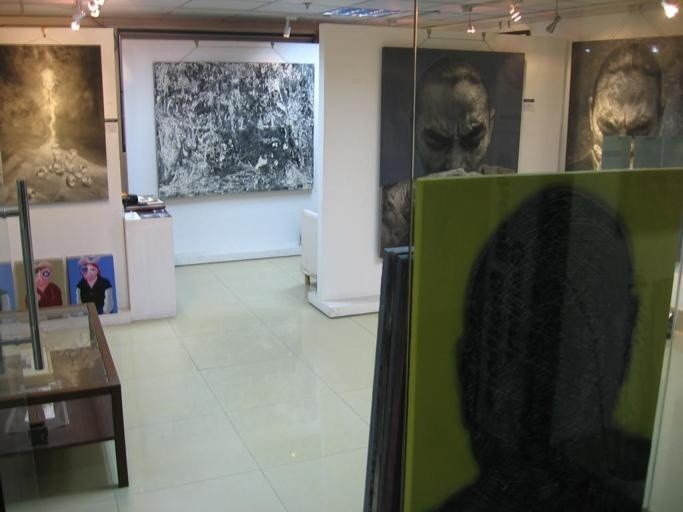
[66,0,101,33]
[545,1,563,35]
[282,15,300,39]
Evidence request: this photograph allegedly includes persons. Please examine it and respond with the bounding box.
[426,184,649,512]
[74,257,113,315]
[383,58,514,247]
[24,262,63,309]
[567,42,665,171]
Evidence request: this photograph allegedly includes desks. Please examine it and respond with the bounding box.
[1,299,129,511]
